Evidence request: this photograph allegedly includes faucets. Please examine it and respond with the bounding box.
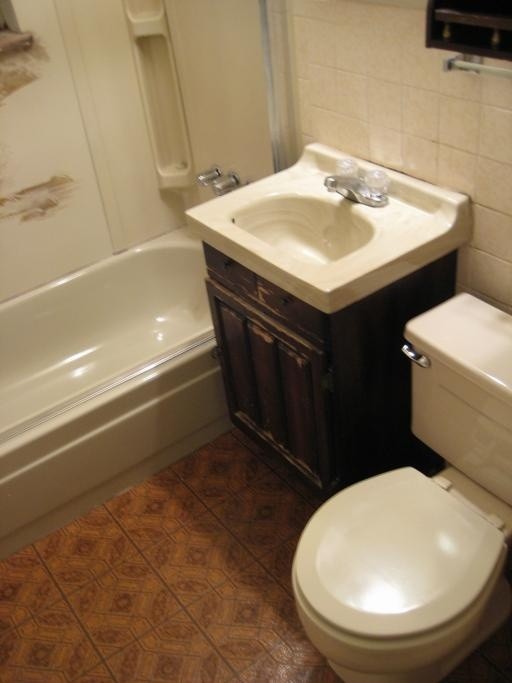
[320,170,393,206]
[207,173,241,196]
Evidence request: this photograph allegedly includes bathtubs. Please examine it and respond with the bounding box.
[0,228,237,565]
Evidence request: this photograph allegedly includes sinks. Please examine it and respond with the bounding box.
[184,140,471,314]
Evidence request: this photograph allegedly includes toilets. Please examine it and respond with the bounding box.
[289,293,511,683]
